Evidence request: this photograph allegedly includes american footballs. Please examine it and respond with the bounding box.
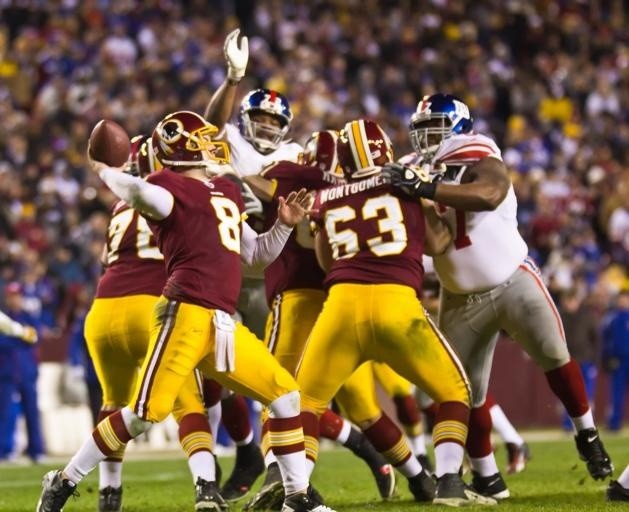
[88,118,132,168]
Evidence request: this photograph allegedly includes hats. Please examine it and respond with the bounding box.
[5,282,23,295]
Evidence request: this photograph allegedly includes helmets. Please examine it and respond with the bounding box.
[405,91,475,156]
[301,127,345,180]
[123,131,160,177]
[236,88,295,156]
[151,111,231,170]
[337,118,395,178]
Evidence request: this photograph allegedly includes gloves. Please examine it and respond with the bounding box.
[379,155,436,202]
[223,28,251,84]
[19,325,38,343]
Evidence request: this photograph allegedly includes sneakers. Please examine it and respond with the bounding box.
[605,479,629,503]
[35,469,80,512]
[194,476,229,512]
[429,471,498,507]
[98,482,123,512]
[505,439,531,476]
[369,451,397,502]
[216,437,266,501]
[467,469,511,501]
[0,454,34,466]
[31,454,51,466]
[571,426,615,481]
[406,454,438,502]
[239,482,335,512]
